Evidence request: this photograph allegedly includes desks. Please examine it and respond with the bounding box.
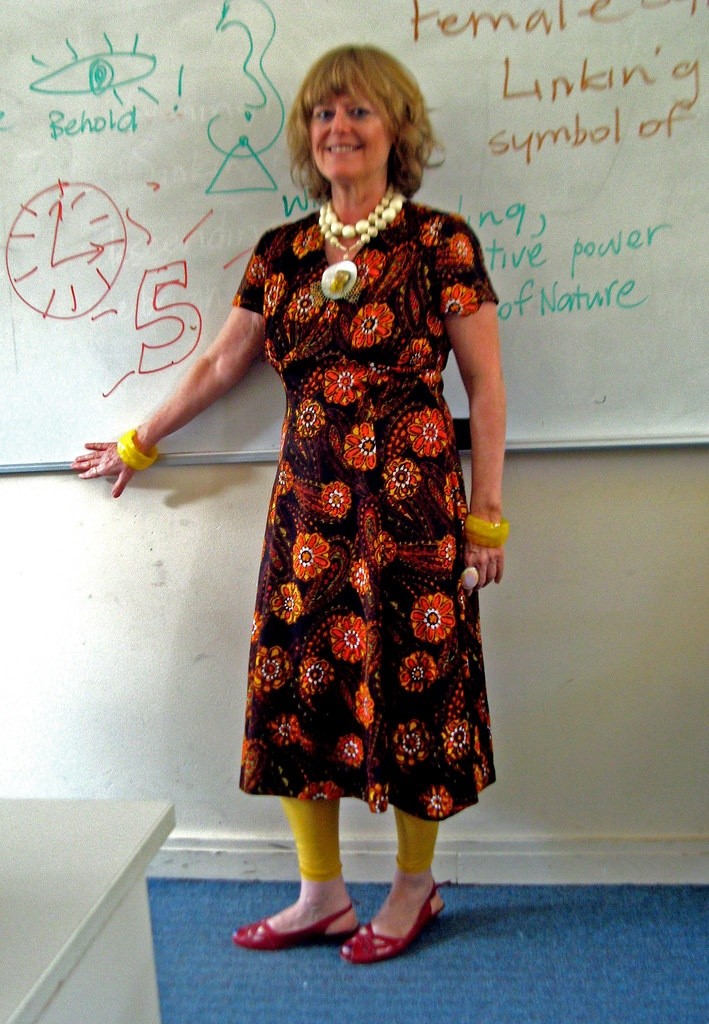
[1,799,178,1024]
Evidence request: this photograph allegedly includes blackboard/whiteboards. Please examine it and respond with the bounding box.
[0,0,709,478]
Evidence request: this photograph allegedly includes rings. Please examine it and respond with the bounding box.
[461,567,478,589]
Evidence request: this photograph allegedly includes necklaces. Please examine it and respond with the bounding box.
[318,184,403,299]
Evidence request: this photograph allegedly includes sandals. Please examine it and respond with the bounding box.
[339,878,444,963]
[232,903,359,950]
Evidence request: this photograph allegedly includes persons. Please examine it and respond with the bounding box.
[70,44,510,962]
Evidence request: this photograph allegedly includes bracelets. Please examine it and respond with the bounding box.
[117,429,157,470]
[465,514,509,547]
